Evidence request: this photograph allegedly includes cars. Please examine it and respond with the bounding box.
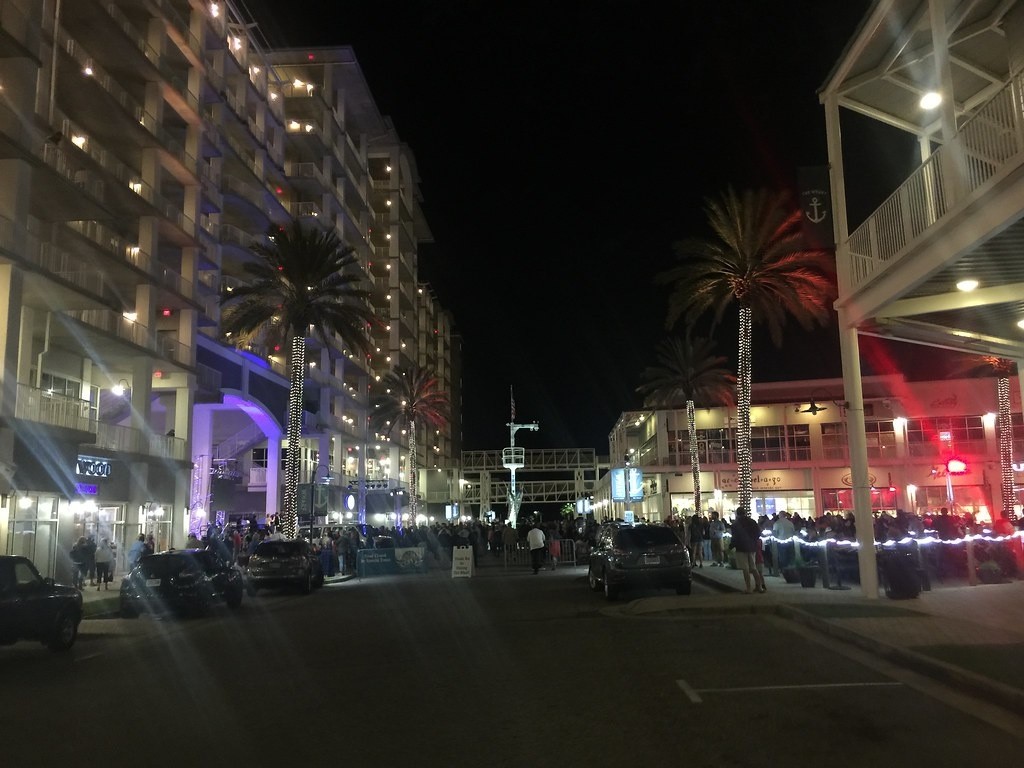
[120,548,244,619]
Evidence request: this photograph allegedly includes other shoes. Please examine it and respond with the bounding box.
[719,564,724,567]
[754,586,764,593]
[699,564,704,568]
[745,588,751,593]
[710,562,718,567]
[533,571,539,575]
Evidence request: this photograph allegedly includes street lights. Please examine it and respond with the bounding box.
[311,464,335,544]
[799,387,879,598]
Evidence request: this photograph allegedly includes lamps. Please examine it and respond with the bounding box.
[148,498,164,515]
[2,484,32,509]
[800,386,850,416]
[112,379,131,396]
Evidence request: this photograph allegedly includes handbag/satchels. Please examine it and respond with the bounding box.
[70,550,77,558]
[108,573,113,582]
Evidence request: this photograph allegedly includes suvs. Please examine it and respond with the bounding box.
[589,522,692,599]
[248,539,323,597]
[0,555,84,651]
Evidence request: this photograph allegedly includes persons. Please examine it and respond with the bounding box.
[128,532,155,575]
[516,505,1024,596]
[221,511,516,579]
[70,532,113,592]
